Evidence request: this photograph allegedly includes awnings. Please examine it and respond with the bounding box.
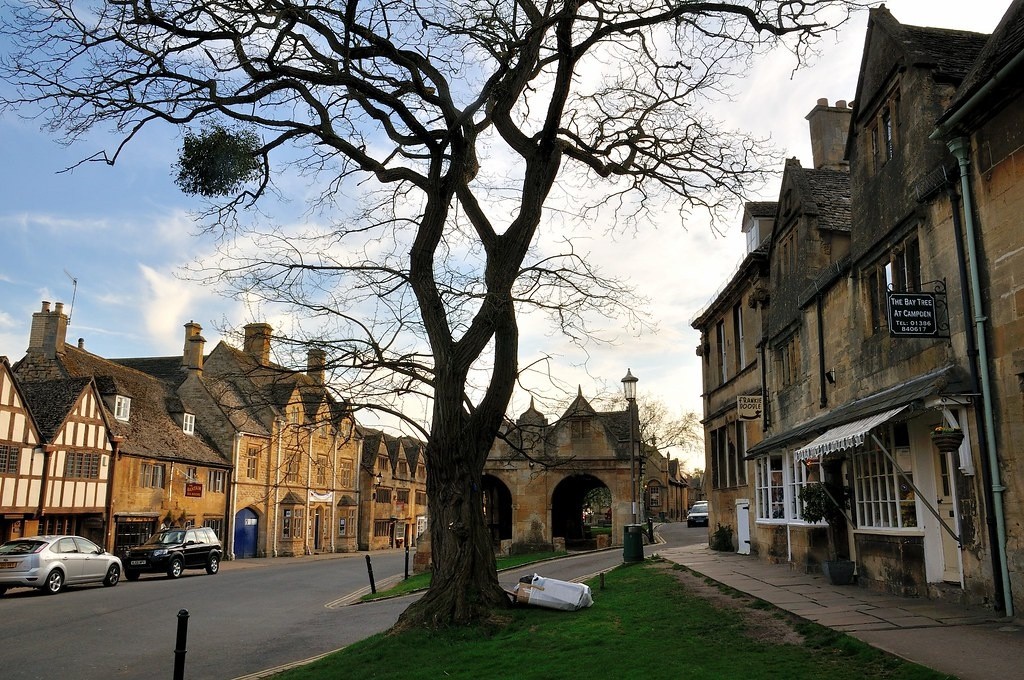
[794,405,962,543]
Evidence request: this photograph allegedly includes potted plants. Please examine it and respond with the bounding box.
[797,483,855,586]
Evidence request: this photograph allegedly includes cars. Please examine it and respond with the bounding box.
[0,534,123,594]
[686,500,709,528]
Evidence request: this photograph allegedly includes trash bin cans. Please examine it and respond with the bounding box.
[622,524,644,561]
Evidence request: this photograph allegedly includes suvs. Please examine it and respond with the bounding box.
[122,524,224,581]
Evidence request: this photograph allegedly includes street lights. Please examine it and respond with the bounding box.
[621,368,640,527]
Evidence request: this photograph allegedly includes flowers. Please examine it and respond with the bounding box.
[930,426,964,436]
